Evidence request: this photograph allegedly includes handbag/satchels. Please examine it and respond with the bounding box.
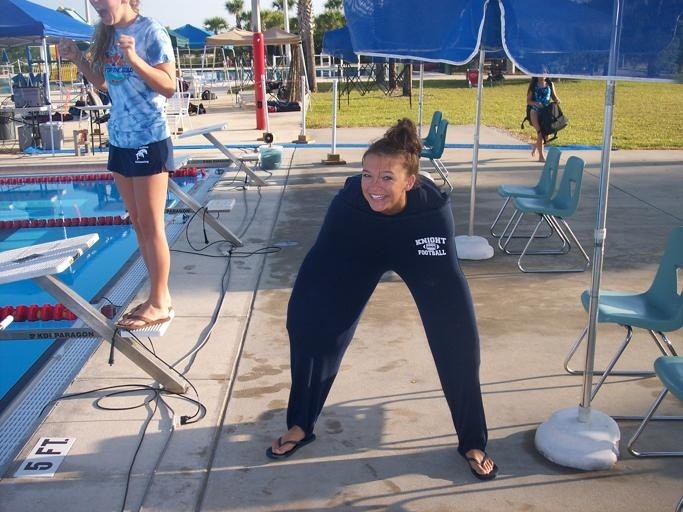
[538,102,569,134]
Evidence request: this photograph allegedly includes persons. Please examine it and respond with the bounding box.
[526,64,561,162]
[404,256,417,259]
[59,0,177,331]
[267,118,499,481]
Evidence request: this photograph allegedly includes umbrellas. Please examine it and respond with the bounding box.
[345,0,682,423]
[323,26,507,142]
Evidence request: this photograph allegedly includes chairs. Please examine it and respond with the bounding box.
[627,356,682,512]
[504,155,591,273]
[164,92,191,135]
[490,146,566,252]
[420,111,449,181]
[420,119,454,194]
[563,226,683,421]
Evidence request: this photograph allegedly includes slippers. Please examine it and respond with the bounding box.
[456,443,501,481]
[115,300,174,329]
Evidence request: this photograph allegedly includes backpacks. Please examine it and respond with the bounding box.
[264,429,317,461]
